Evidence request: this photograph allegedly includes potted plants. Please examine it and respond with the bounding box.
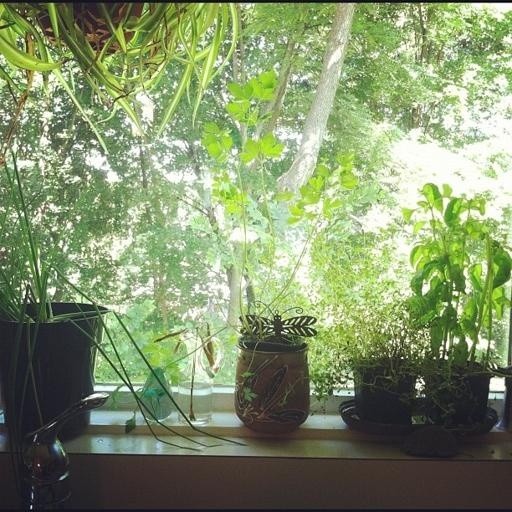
[0,2,244,134]
[340,298,420,427]
[228,297,314,434]
[398,183,512,427]
[1,146,246,453]
